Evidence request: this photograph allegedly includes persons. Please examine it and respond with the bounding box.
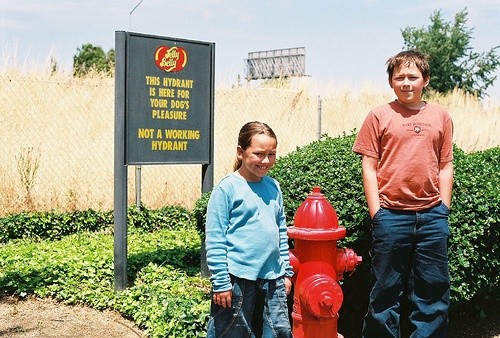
[204,121,295,338]
[352,51,454,337]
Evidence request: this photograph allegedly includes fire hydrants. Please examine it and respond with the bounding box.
[286,187,362,338]
